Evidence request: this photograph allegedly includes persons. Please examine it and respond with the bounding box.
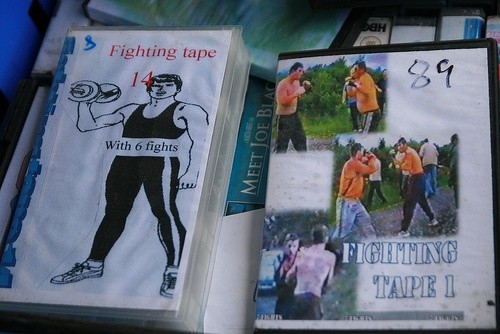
[273,62,313,154]
[274,223,336,320]
[343,61,388,132]
[331,134,460,241]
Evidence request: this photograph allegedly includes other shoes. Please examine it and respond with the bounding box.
[395,230,411,237]
[426,219,439,226]
[381,200,386,204]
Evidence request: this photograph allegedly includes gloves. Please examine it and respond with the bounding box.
[303,81,312,91]
